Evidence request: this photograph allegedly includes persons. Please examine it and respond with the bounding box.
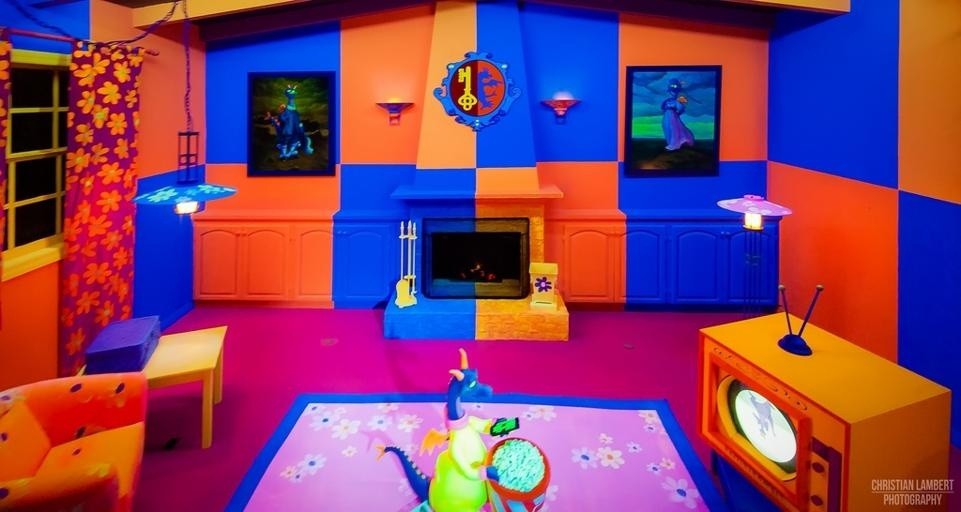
[373,346,553,511]
